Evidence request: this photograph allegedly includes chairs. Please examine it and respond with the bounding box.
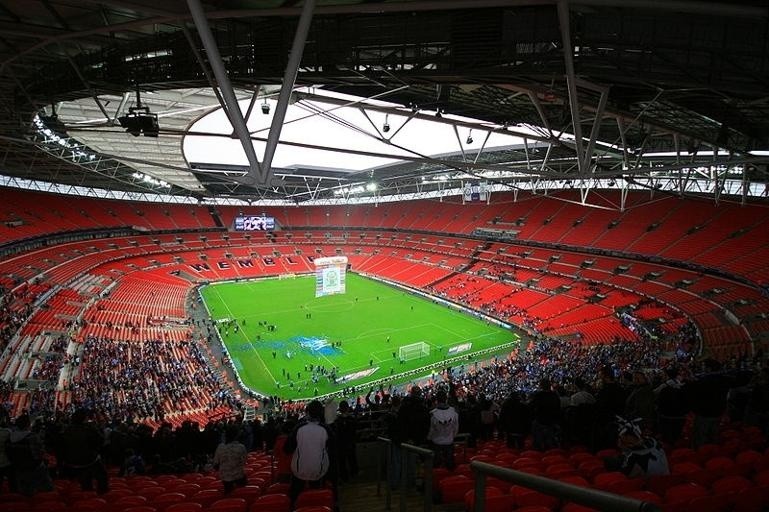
[0,432,769,512]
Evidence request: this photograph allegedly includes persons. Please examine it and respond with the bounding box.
[1,248,768,512]
